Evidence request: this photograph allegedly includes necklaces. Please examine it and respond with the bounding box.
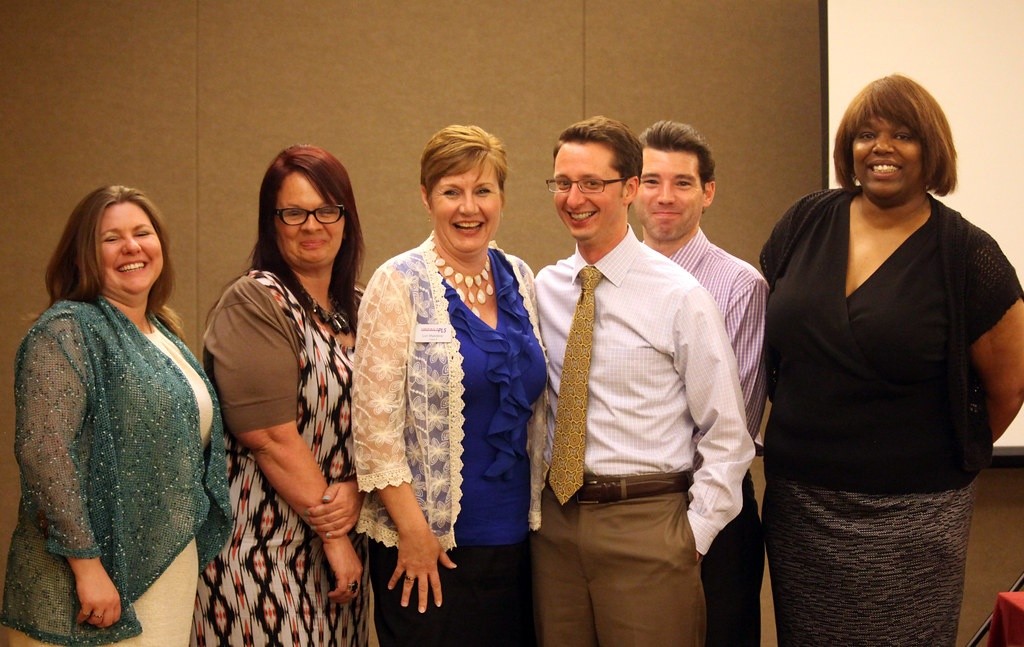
[307,295,351,335]
[436,255,493,319]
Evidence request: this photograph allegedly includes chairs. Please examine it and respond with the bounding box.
[967,575,1024,647]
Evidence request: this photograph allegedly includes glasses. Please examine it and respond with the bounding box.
[276,205,344,226]
[546,178,628,193]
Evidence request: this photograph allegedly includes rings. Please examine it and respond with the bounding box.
[405,575,413,581]
[81,613,89,617]
[348,581,358,594]
[92,615,103,618]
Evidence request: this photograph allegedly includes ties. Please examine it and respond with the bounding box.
[549,266,603,506]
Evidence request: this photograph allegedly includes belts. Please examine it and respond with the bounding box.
[543,476,689,504]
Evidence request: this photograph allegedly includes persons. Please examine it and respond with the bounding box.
[190,144,367,647]
[633,121,773,647]
[0,184,233,647]
[351,125,551,647]
[759,75,1024,647]
[531,115,755,647]
